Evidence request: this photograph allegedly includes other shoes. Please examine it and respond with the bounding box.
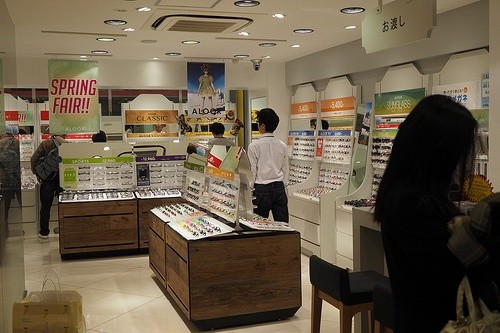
[38,233,50,239]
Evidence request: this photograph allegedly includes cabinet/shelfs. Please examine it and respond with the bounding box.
[18,95,500,333]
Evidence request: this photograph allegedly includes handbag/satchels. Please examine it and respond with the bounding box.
[12,268,86,333]
[440,276,500,333]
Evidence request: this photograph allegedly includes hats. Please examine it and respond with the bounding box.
[12,126,19,134]
[6,126,13,134]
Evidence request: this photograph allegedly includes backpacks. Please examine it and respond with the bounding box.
[0,138,21,184]
[33,138,63,183]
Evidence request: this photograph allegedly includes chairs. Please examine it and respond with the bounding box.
[310,254,392,333]
[370,282,392,333]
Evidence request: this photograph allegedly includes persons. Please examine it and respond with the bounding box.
[367,94,480,333]
[206,121,235,147]
[0,125,33,232]
[247,108,289,223]
[197,63,218,112]
[30,134,69,243]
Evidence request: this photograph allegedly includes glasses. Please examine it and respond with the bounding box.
[345,198,375,207]
[256,120,259,124]
[186,179,201,202]
[285,137,351,199]
[150,163,187,184]
[156,201,194,217]
[137,189,181,197]
[61,190,134,201]
[178,215,221,237]
[78,165,133,186]
[371,173,382,198]
[209,180,238,218]
[239,215,284,228]
[371,138,393,165]
[56,135,65,139]
